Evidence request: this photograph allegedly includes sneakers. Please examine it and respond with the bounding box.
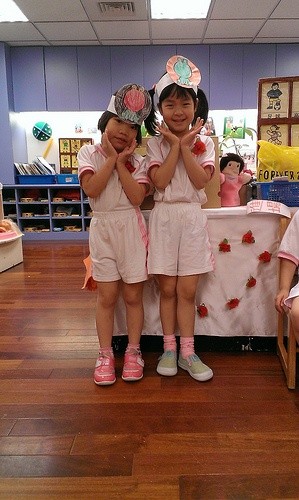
[121,348,144,381]
[178,353,213,381]
[93,351,116,385]
[156,350,177,376]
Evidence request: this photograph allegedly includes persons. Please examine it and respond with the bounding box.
[143,72,215,381]
[78,90,149,385]
[274,211,299,342]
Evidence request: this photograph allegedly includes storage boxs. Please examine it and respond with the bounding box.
[16,173,80,184]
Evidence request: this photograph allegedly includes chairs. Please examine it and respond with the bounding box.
[276,208,299,389]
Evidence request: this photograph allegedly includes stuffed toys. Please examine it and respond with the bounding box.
[220,153,251,206]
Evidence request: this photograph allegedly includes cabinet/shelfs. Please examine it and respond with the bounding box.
[0,230,24,274]
[2,183,93,240]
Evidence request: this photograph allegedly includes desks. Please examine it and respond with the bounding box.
[80,205,299,353]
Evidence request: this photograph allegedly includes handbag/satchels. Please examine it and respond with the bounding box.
[256,140,299,182]
